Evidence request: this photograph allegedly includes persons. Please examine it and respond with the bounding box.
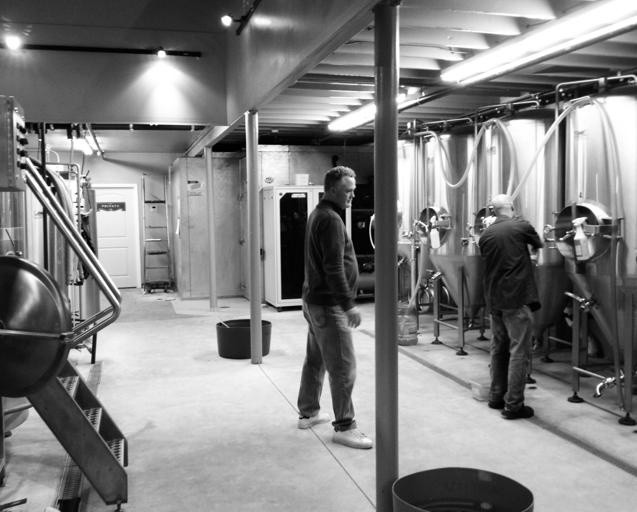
[298,166,374,449]
[479,193,544,419]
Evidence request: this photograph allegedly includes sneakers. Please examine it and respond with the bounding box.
[297,413,331,429]
[488,399,506,409]
[501,406,534,420]
[332,428,373,449]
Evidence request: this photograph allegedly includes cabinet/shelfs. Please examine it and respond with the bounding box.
[261,187,352,310]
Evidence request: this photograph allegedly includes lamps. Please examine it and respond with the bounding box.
[221,0,263,35]
[1,35,203,60]
